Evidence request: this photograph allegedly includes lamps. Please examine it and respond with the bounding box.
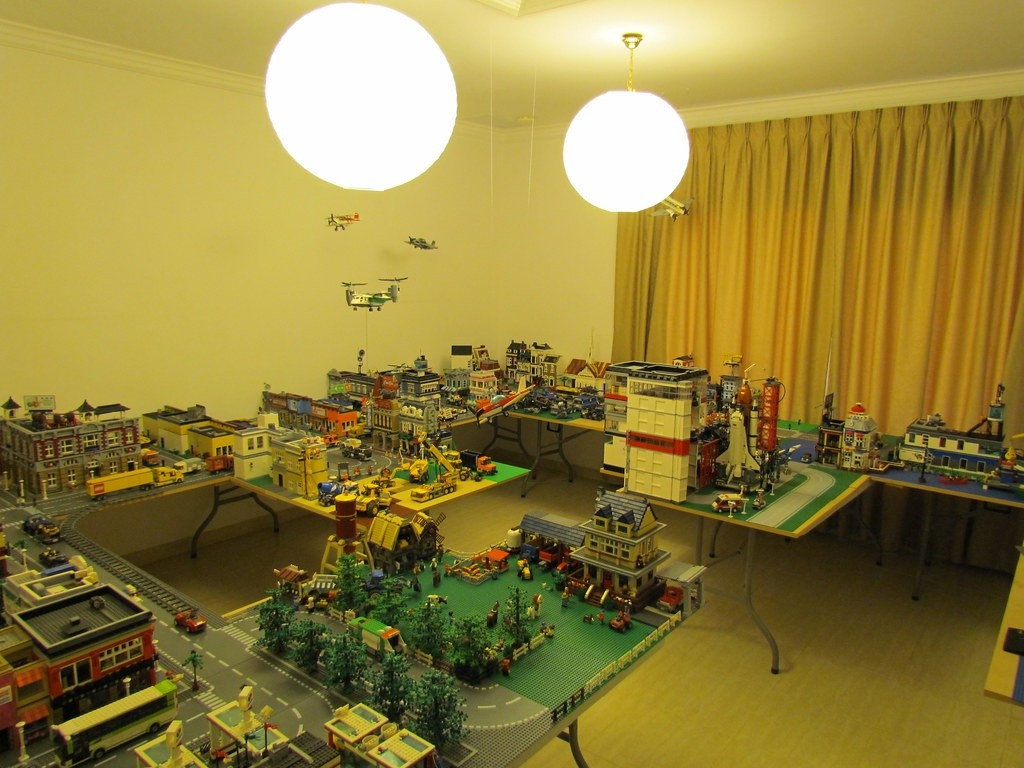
[264,1,458,191]
[562,33,690,212]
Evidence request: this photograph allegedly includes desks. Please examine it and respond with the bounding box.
[0,374,1023,767]
